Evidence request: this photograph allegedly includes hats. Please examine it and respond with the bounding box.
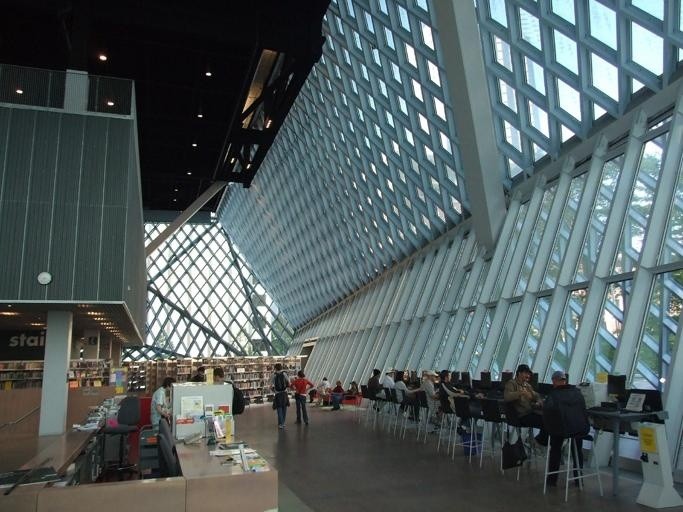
[552,371,565,379]
[427,370,438,376]
[516,365,533,377]
[385,367,394,373]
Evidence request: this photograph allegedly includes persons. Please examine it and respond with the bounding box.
[270,364,295,429]
[149,378,175,437]
[312,380,343,408]
[503,364,552,448]
[309,376,330,403]
[290,371,314,424]
[330,381,359,411]
[365,368,475,436]
[534,370,588,487]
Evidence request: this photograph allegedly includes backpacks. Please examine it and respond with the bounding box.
[224,381,245,414]
[274,371,286,391]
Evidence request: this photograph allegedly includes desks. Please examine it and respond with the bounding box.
[584,407,669,496]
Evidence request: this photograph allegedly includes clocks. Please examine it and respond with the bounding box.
[36,271,53,285]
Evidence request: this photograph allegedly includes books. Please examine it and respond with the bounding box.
[77,400,120,430]
[1,361,44,390]
[0,467,63,490]
[69,359,110,390]
[122,361,177,393]
[180,395,203,417]
[177,359,294,396]
[204,404,271,475]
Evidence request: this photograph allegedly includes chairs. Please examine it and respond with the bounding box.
[359,371,605,501]
[155,417,179,478]
[308,389,363,410]
[100,396,142,478]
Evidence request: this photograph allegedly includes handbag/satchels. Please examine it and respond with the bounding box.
[503,439,526,470]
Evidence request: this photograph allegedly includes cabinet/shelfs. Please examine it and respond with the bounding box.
[0,359,106,388]
[124,357,305,400]
